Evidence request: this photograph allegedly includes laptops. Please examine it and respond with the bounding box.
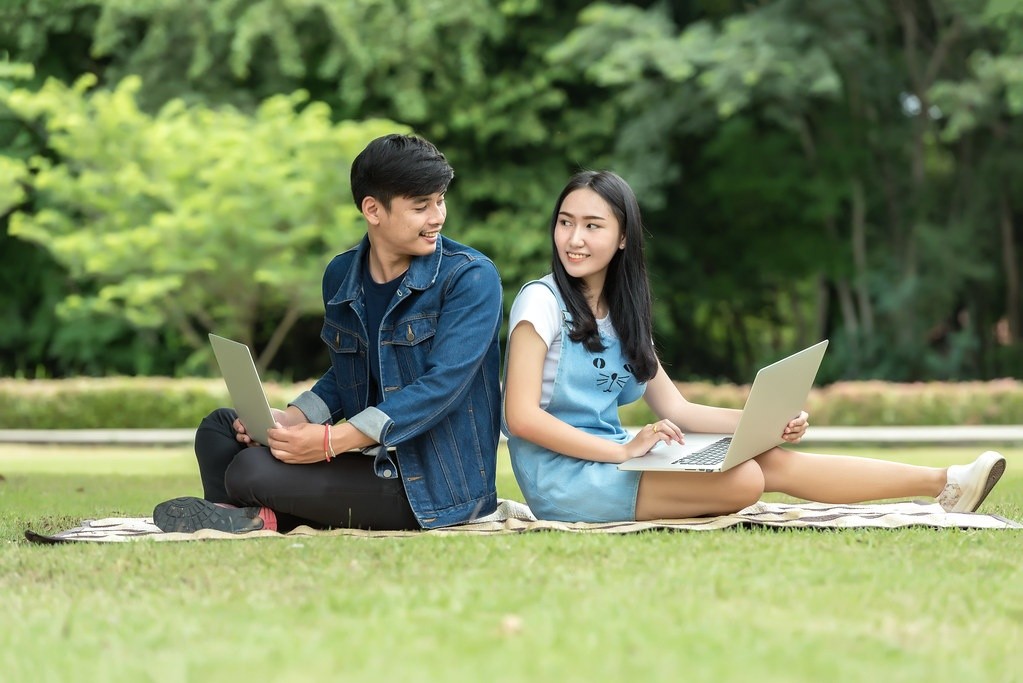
[207,332,397,455]
[616,338,831,474]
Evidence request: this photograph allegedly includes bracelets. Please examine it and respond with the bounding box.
[324,423,330,462]
[328,425,335,458]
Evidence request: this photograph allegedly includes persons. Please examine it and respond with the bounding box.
[153,134,503,534]
[500,170,1006,523]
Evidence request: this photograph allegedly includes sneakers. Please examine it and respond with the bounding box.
[934,451,1006,513]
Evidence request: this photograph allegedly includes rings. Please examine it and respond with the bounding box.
[654,426,657,433]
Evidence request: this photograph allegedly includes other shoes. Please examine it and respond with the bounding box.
[153,497,278,533]
